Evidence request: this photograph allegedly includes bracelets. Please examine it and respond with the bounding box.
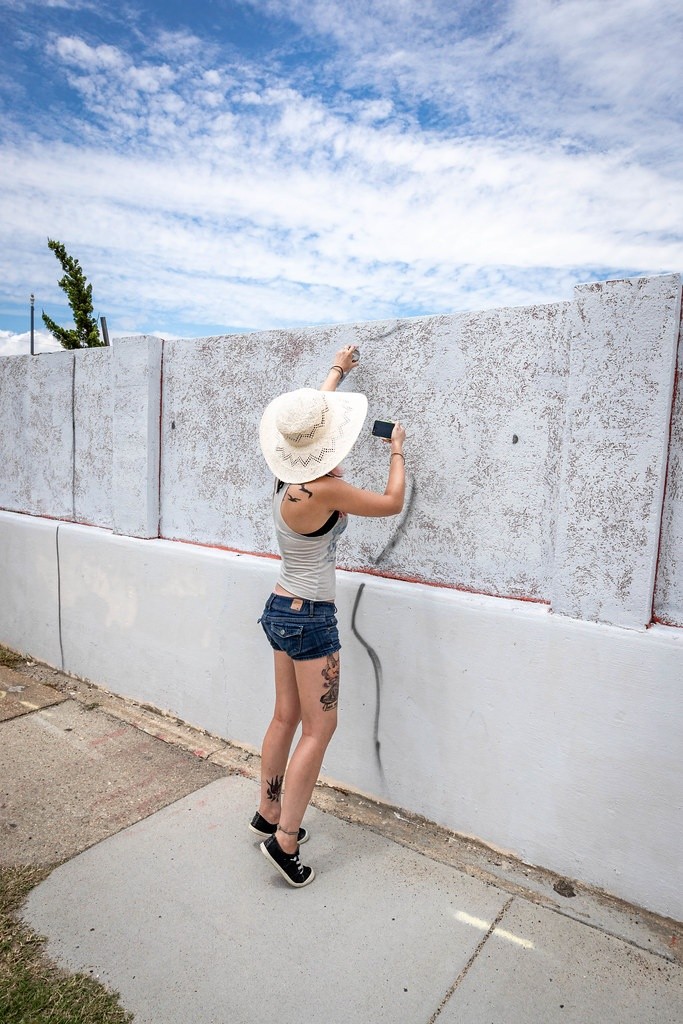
[390,453,405,466]
[330,366,343,379]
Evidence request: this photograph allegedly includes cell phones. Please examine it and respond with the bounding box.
[372,420,395,438]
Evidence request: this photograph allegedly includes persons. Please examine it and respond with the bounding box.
[249,345,406,888]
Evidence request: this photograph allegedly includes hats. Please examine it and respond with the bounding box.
[259,388,368,484]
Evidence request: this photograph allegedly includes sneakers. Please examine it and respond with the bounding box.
[260,833,315,888]
[248,811,309,845]
